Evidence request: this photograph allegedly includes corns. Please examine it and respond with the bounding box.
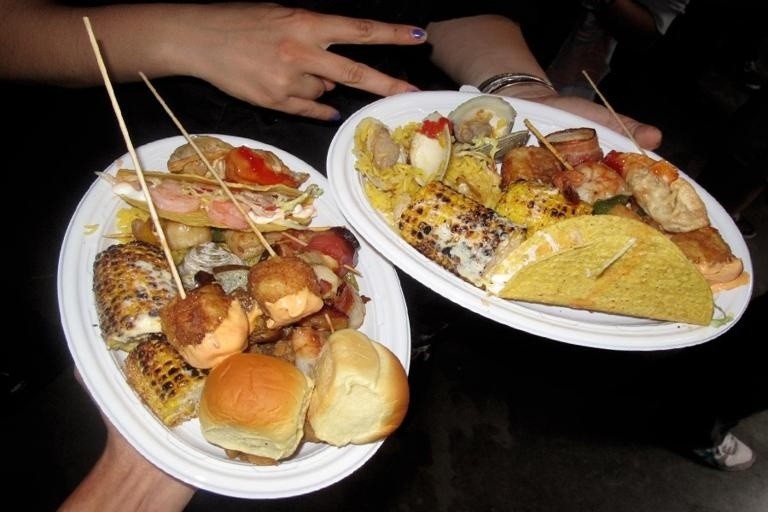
[494,178,594,240]
[93,240,178,353]
[399,181,526,289]
[124,332,209,427]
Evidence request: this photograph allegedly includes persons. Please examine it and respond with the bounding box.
[671,291,768,471]
[0,0,430,124]
[53,362,200,511]
[415,1,663,152]
[688,89,767,240]
[576,1,688,40]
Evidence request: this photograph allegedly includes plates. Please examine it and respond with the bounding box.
[326,90,755,352]
[56,132,412,499]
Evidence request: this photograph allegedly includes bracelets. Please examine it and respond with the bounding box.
[582,0,615,15]
[473,70,552,100]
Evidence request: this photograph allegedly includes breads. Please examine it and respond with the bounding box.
[306,328,411,449]
[198,353,310,461]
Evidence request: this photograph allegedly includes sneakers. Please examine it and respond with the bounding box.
[689,432,756,472]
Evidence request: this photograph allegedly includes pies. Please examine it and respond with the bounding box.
[485,214,715,327]
